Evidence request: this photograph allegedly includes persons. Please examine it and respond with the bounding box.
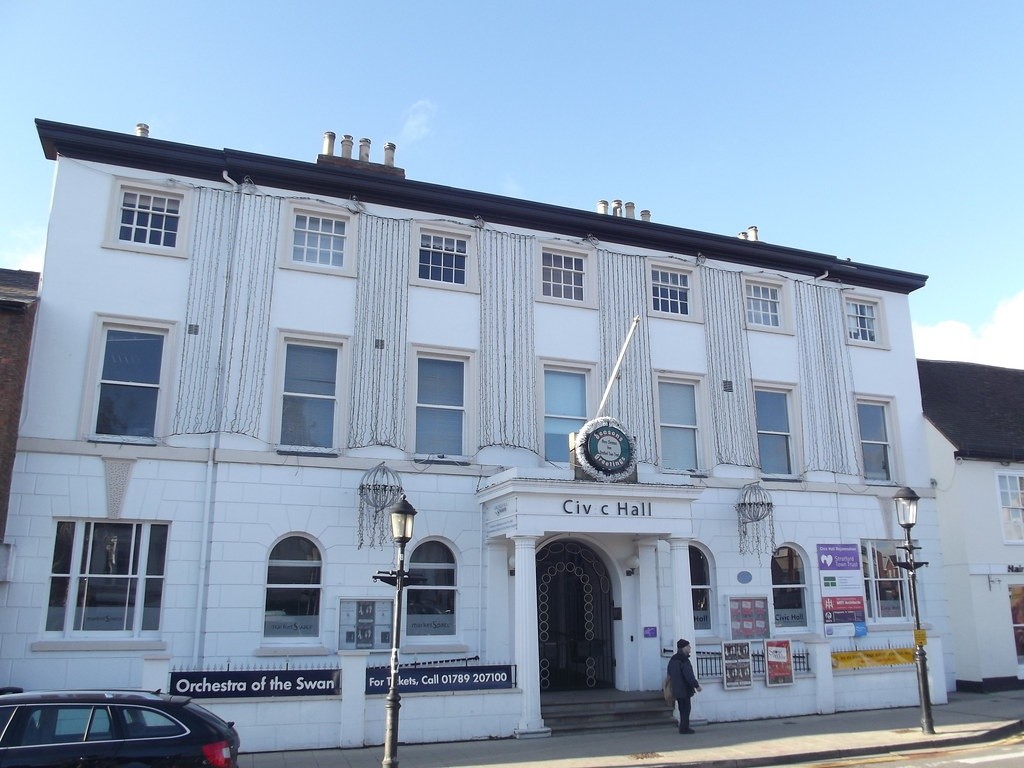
[667,639,702,735]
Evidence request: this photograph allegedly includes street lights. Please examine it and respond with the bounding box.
[371,493,427,768]
[892,486,936,736]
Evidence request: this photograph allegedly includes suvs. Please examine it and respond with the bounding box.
[0,686,240,768]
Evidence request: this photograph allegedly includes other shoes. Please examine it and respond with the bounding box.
[679,728,695,734]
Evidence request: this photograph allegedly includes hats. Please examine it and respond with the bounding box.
[677,639,689,649]
